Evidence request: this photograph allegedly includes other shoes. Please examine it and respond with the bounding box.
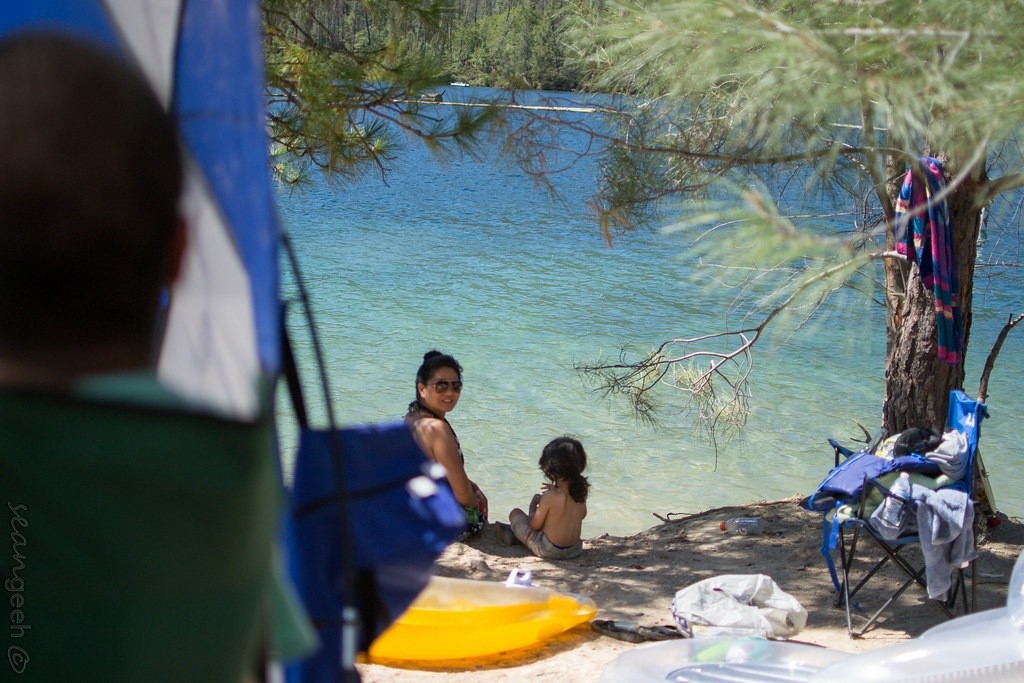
[495,521,521,547]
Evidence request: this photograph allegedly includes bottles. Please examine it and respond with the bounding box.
[878,472,910,541]
[719,517,765,534]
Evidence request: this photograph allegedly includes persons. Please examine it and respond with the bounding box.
[0,31,320,683]
[495,438,589,559]
[403,351,488,541]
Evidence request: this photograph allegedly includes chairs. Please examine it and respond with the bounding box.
[820,391,989,638]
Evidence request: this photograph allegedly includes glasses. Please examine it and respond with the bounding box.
[425,380,462,393]
